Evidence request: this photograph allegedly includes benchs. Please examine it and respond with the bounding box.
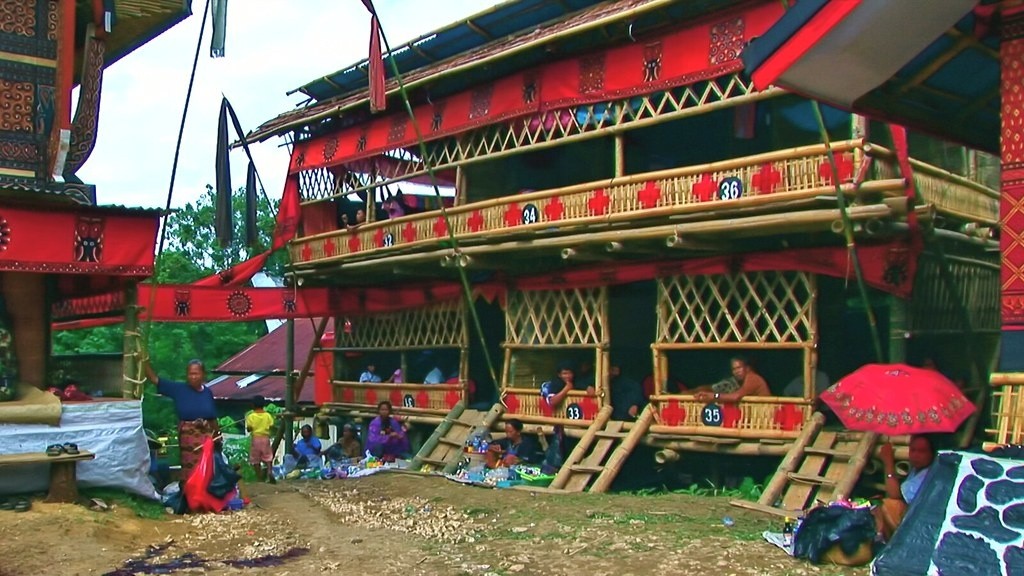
[0,450,95,504]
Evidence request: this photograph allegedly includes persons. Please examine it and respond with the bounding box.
[245,394,414,485]
[144,358,224,515]
[540,355,771,422]
[487,418,540,469]
[360,359,477,399]
[342,210,365,228]
[878,434,936,531]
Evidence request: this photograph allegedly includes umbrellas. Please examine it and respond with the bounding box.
[819,362,977,435]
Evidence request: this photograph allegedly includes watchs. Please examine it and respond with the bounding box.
[885,473,896,478]
[714,392,719,401]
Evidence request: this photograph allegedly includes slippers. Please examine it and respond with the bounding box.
[1,496,18,510]
[63,442,80,455]
[14,496,32,511]
[46,443,62,456]
